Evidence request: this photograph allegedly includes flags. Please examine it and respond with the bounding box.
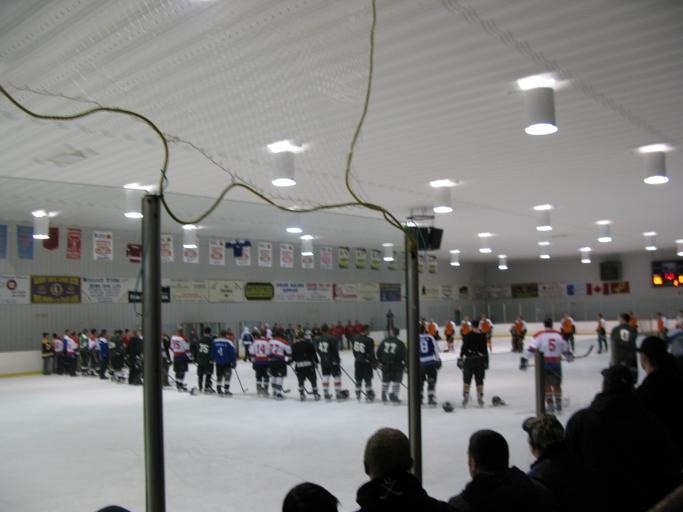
[441,279,631,304]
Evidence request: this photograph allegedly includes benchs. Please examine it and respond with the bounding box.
[648,483,682,511]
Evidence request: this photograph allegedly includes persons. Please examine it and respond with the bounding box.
[609,312,638,384]
[558,311,576,351]
[674,309,682,329]
[1,224,441,305]
[655,311,668,339]
[593,311,609,354]
[273,334,682,512]
[628,310,639,338]
[40,310,527,407]
[518,316,574,412]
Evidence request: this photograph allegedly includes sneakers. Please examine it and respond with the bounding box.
[443,348,609,355]
[300,392,305,400]
[356,393,361,400]
[381,395,388,404]
[42,370,232,394]
[390,394,400,405]
[324,395,333,402]
[462,398,486,408]
[548,405,564,412]
[365,393,374,402]
[336,393,347,400]
[257,391,284,398]
[313,394,320,400]
[428,395,437,405]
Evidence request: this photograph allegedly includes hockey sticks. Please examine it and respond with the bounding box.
[527,345,593,366]
[233,367,248,393]
[281,388,289,393]
[289,363,314,394]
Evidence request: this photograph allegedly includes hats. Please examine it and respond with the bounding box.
[364,429,414,472]
[636,336,669,353]
[602,362,639,387]
[522,414,568,457]
